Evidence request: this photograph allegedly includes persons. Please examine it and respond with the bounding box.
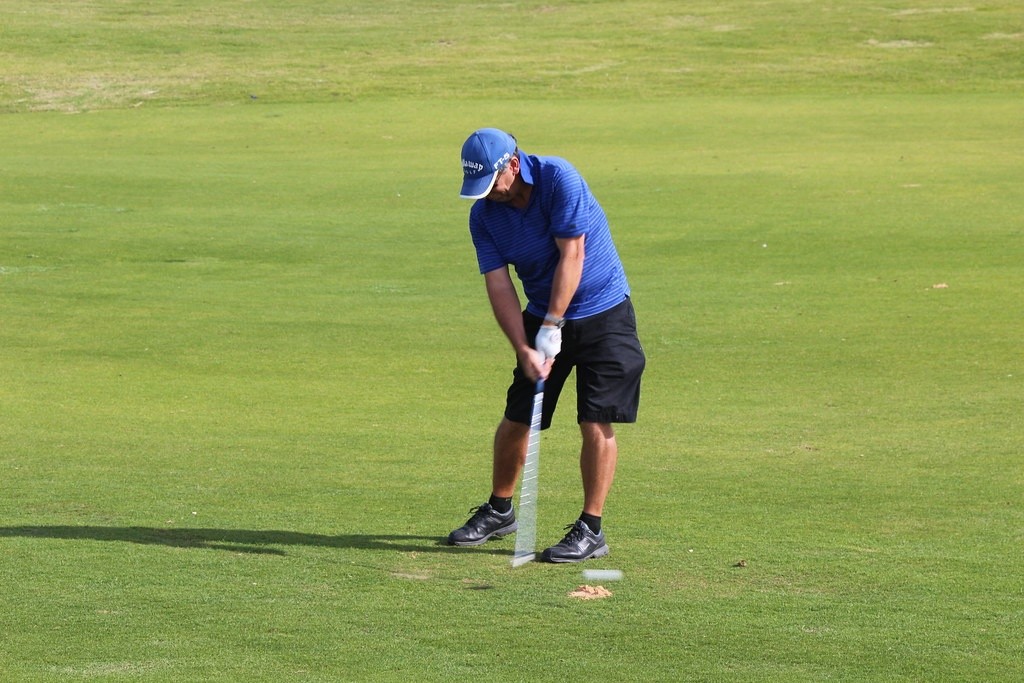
[448,127,646,563]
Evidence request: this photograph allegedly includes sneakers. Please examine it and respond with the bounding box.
[541,520,609,562]
[447,502,517,545]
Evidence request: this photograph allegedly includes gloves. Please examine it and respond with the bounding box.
[534,315,562,365]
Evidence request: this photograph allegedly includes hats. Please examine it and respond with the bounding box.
[459,128,516,200]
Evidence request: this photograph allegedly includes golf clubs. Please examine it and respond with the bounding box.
[511,376,546,568]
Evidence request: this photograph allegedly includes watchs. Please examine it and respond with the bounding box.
[545,313,565,328]
